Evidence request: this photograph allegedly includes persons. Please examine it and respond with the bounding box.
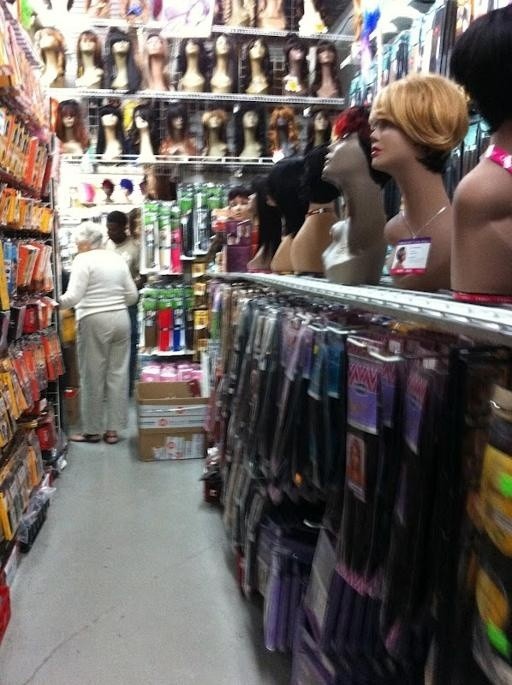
[394,247,406,268]
[141,33,175,91]
[289,141,340,274]
[368,71,470,290]
[321,103,392,286]
[158,103,200,160]
[129,105,160,162]
[200,105,229,162]
[104,210,147,397]
[347,438,363,486]
[35,27,66,88]
[55,99,90,159]
[102,180,115,205]
[102,31,141,91]
[119,179,133,203]
[57,221,139,444]
[243,35,269,94]
[311,40,341,99]
[209,32,237,93]
[246,172,282,271]
[176,37,211,91]
[448,1,512,296]
[268,106,299,162]
[228,186,249,222]
[96,106,128,161]
[265,154,304,273]
[303,103,331,155]
[280,33,306,95]
[75,30,104,89]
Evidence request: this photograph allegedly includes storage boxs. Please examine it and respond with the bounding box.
[133,380,210,462]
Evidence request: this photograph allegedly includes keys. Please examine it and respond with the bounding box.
[234,103,265,163]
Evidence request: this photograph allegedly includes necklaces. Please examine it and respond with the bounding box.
[304,207,335,218]
[484,143,512,175]
[401,204,448,239]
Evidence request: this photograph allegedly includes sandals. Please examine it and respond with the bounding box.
[102,430,119,443]
[68,430,101,444]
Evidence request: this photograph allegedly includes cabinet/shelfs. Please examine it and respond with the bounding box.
[209,271,511,679]
[0,2,71,640]
[32,2,356,419]
